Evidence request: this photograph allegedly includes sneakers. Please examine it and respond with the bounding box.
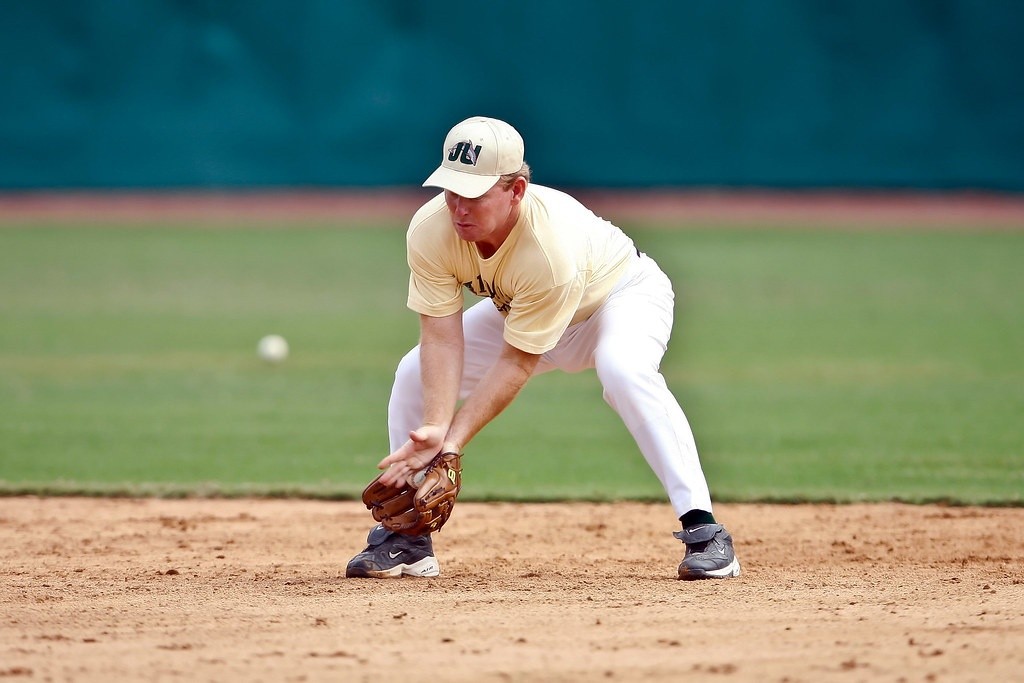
[346,524,440,579]
[673,523,740,579]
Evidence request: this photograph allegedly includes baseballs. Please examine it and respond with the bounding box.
[255,334,289,367]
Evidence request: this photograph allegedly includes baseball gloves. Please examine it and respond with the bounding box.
[361,451,462,537]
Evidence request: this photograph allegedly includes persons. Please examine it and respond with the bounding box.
[345,115,740,583]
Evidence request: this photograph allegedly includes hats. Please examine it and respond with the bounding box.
[421,115,524,199]
